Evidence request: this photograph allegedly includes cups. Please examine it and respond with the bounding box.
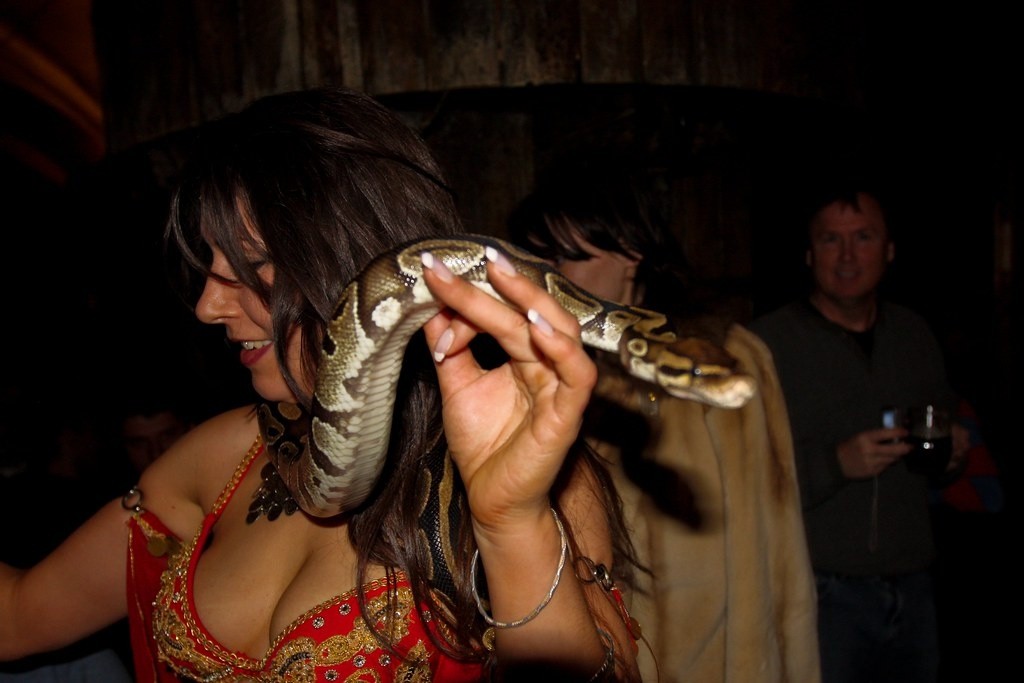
[904,406,954,476]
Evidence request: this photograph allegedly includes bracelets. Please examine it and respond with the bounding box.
[591,628,614,683]
[468,507,570,627]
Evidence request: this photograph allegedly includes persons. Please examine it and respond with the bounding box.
[0,86,1024,683]
